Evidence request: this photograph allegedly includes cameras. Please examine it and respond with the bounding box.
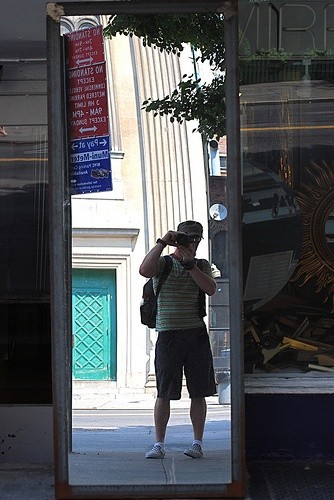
[176,233,188,246]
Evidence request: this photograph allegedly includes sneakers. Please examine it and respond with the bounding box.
[145,446,166,459]
[184,443,204,458]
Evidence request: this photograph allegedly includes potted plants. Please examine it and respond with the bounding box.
[241,48,305,85]
[300,47,334,81]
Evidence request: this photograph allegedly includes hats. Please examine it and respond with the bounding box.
[177,221,204,239]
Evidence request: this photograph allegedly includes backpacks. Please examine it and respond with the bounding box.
[140,255,207,329]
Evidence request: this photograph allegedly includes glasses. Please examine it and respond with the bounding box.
[189,236,201,243]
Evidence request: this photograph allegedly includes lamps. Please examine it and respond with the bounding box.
[210,263,221,279]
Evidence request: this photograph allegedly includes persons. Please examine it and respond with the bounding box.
[139,221,217,459]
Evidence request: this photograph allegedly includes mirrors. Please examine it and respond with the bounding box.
[45,0,247,499]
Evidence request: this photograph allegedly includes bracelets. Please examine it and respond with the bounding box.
[180,257,199,271]
[156,238,167,247]
